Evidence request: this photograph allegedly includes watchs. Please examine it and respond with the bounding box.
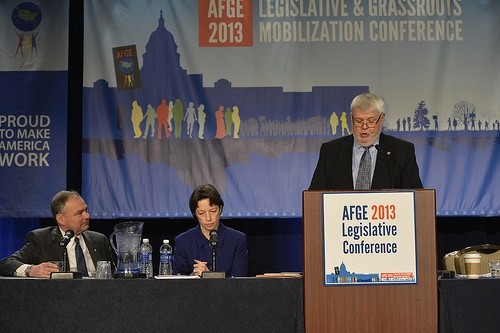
[25,266,33,277]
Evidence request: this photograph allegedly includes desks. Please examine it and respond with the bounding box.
[0,275,306,333]
[438,277,500,333]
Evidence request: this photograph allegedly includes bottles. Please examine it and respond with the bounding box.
[159,239,173,276]
[139,239,153,277]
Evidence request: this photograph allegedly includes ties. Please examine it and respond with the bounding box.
[354,146,371,190]
[74,238,88,277]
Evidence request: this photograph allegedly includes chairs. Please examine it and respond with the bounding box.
[442,244,500,275]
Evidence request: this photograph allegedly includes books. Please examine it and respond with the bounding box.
[154,275,201,279]
[256,272,304,278]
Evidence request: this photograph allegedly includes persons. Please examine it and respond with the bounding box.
[308,92,424,190]
[0,190,117,278]
[171,184,248,277]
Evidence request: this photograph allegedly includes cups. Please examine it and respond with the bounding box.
[48,261,63,272]
[488,260,500,279]
[95,261,112,279]
[464,254,481,279]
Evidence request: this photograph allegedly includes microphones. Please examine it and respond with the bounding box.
[210,229,219,246]
[59,230,74,247]
[375,144,393,188]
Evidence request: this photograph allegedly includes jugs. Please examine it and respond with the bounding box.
[110,221,144,278]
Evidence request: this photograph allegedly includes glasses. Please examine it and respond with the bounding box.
[351,114,381,128]
